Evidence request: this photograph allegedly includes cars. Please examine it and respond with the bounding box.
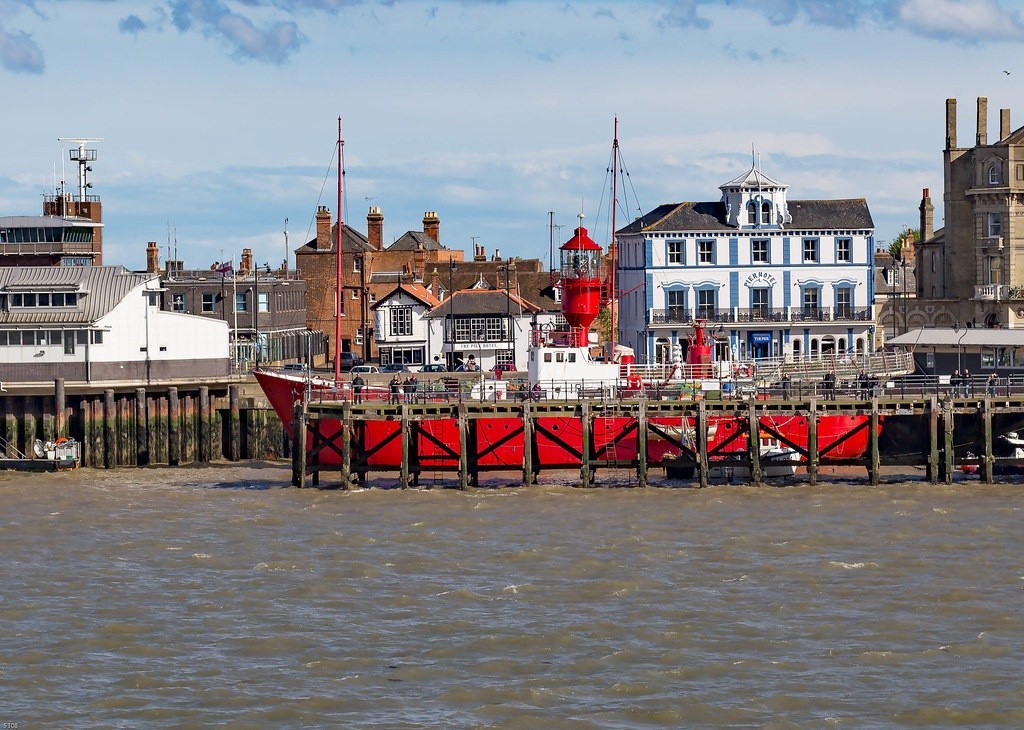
[348,366,380,375]
[454,365,483,372]
[284,363,308,373]
[488,364,517,371]
[417,365,449,373]
[382,363,411,373]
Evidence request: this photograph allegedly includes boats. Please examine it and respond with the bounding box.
[708,433,801,477]
[0,438,81,474]
[661,413,701,480]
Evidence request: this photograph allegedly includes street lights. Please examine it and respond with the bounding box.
[892,257,899,339]
[449,256,458,372]
[253,262,273,373]
[900,256,908,334]
[477,329,484,413]
[953,320,968,398]
[499,260,511,364]
[710,322,725,400]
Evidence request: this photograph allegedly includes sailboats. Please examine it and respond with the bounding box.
[248,110,891,471]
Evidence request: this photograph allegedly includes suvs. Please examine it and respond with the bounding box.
[332,352,365,372]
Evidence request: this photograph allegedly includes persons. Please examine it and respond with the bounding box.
[519,384,541,403]
[403,375,418,404]
[782,373,790,401]
[858,369,878,401]
[961,369,971,398]
[950,370,962,399]
[351,374,365,405]
[989,374,996,398]
[825,370,834,400]
[465,354,483,372]
[389,374,402,406]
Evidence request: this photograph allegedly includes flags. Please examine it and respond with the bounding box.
[215,260,233,272]
